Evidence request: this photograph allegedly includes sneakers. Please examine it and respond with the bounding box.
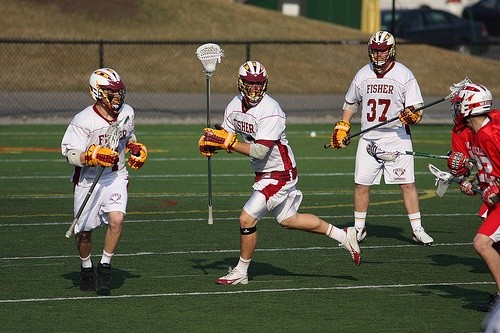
[215,269,248,284]
[411,228,433,246]
[356,227,367,241]
[338,227,362,266]
[96,261,112,295]
[79,261,94,290]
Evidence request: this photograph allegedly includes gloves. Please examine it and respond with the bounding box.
[330,123,351,148]
[460,181,480,196]
[481,177,500,206]
[84,144,119,166]
[197,123,238,156]
[398,105,421,126]
[125,142,147,169]
[447,151,467,177]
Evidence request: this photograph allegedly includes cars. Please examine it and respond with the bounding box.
[380,0,479,21]
[379,8,490,57]
[462,0,500,45]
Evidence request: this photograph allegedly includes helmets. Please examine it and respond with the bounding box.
[88,68,125,116]
[445,77,494,126]
[368,31,395,72]
[237,60,269,106]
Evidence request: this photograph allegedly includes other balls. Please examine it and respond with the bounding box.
[310,131,317,138]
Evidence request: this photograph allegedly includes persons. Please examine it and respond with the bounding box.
[199,61,361,284]
[448,83,500,333]
[331,31,434,243]
[61,68,147,292]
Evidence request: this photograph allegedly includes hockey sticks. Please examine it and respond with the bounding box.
[428,162,500,204]
[323,76,473,151]
[366,139,476,163]
[64,114,132,239]
[194,43,225,225]
